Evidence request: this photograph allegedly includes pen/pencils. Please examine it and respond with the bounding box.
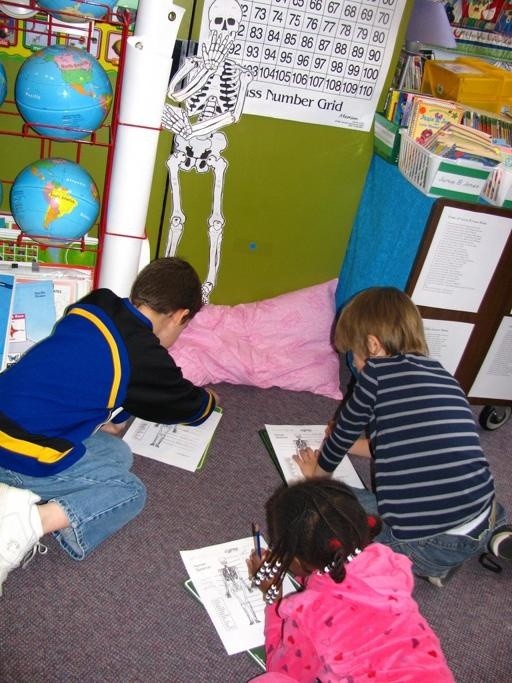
[254,522,261,559]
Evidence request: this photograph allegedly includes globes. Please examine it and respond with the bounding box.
[37,0,118,23]
[10,157,100,247]
[0,64,8,106]
[15,45,113,142]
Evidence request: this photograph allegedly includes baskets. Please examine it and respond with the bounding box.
[398,128,512,209]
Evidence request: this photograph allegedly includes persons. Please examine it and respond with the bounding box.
[0,256,222,597]
[293,287,512,586]
[245,479,455,683]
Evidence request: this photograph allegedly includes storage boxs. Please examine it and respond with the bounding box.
[421,56,511,115]
[373,111,409,163]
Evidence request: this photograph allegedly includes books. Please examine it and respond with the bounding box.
[382,48,512,208]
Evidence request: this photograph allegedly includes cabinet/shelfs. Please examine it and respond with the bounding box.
[330,158,510,431]
[0,1,131,342]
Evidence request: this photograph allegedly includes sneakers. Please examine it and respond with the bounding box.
[0,482,44,598]
[487,527,512,562]
